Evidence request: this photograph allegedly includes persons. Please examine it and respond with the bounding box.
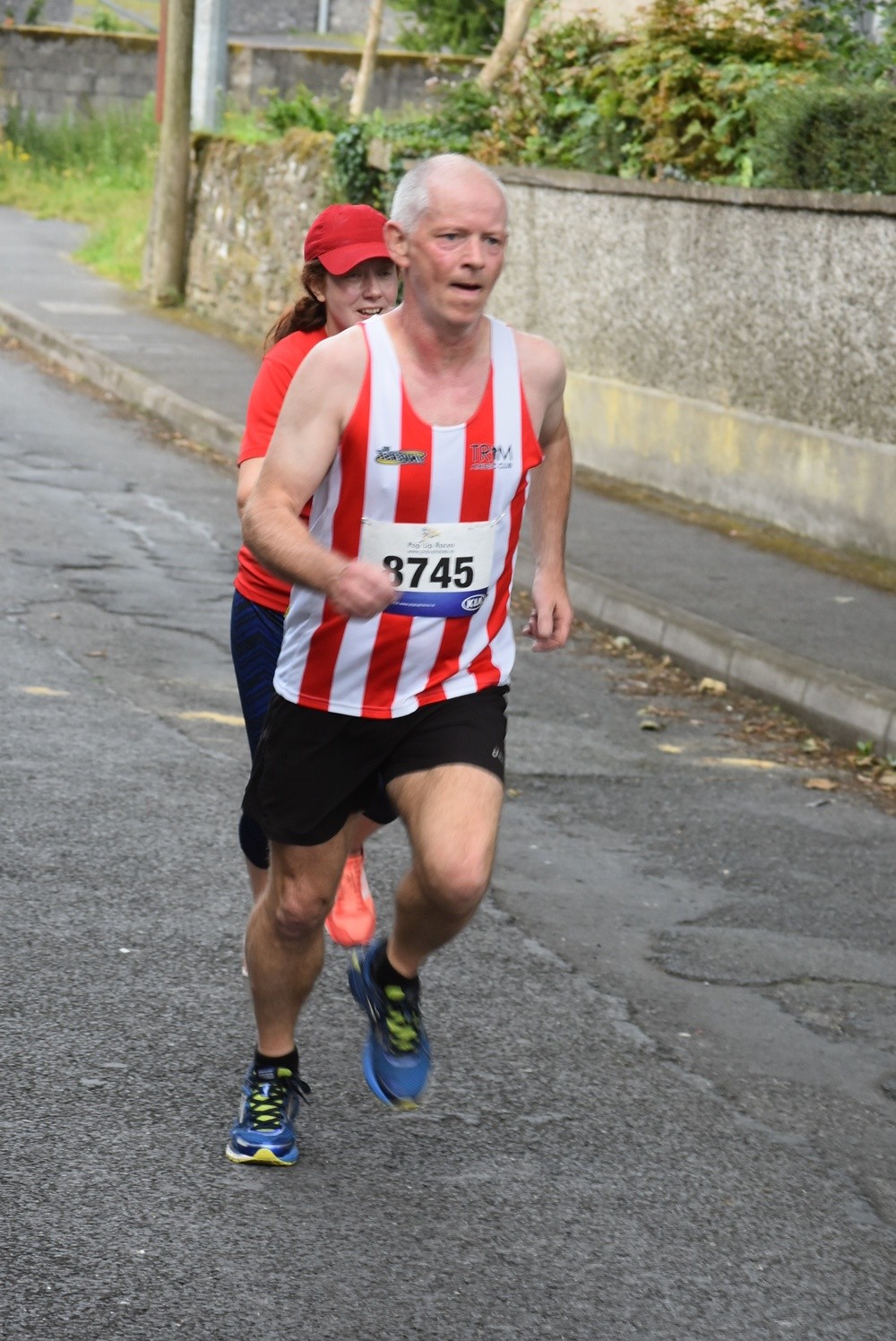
[229,204,377,950]
[225,159,575,1167]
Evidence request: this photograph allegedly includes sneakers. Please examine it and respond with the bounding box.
[325,846,375,948]
[226,1062,311,1166]
[346,936,430,1113]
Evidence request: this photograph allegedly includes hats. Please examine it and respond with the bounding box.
[304,204,391,275]
